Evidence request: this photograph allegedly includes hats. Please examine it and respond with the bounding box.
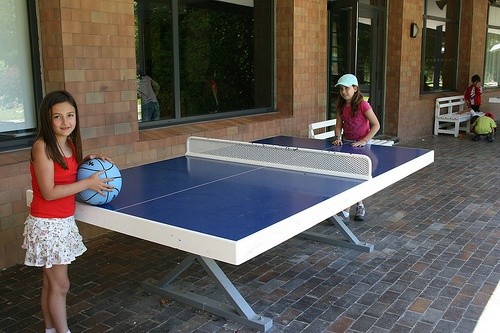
[335,73,358,87]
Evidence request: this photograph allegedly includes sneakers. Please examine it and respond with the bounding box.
[354,206,365,221]
[325,211,350,225]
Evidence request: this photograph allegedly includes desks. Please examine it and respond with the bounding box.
[25,135,434,331]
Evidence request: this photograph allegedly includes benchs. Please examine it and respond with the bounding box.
[309,118,395,152]
[435,95,486,138]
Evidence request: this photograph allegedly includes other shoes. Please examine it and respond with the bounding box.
[470,135,494,142]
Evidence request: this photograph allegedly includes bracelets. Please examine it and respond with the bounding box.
[335,136,342,140]
[361,138,368,142]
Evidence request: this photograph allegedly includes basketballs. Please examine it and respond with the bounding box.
[77,158,123,205]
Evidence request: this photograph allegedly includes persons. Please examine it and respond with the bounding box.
[135,70,161,122]
[331,73,380,222]
[467,107,485,139]
[19,89,115,333]
[464,75,482,134]
[471,111,497,142]
